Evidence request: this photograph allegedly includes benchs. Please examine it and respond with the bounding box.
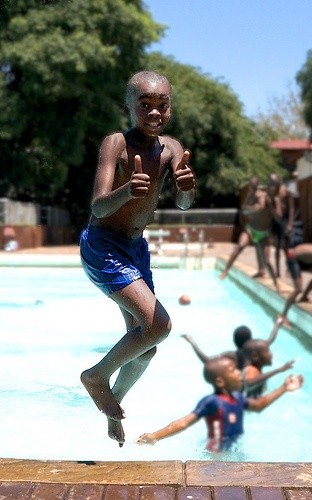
[144,230,170,255]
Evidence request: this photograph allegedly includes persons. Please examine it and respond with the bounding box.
[135,356,305,455]
[179,320,283,368]
[237,340,296,401]
[218,178,283,298]
[267,172,295,276]
[252,182,282,279]
[274,243,312,330]
[78,72,197,449]
